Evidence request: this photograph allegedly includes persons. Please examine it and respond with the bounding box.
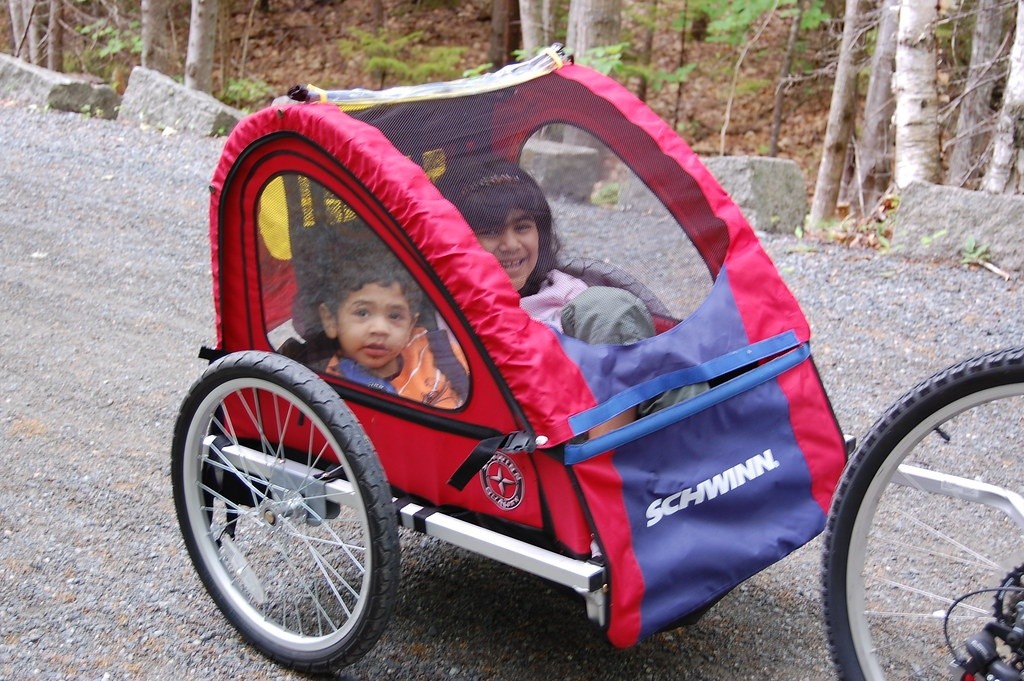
[309,158,711,443]
